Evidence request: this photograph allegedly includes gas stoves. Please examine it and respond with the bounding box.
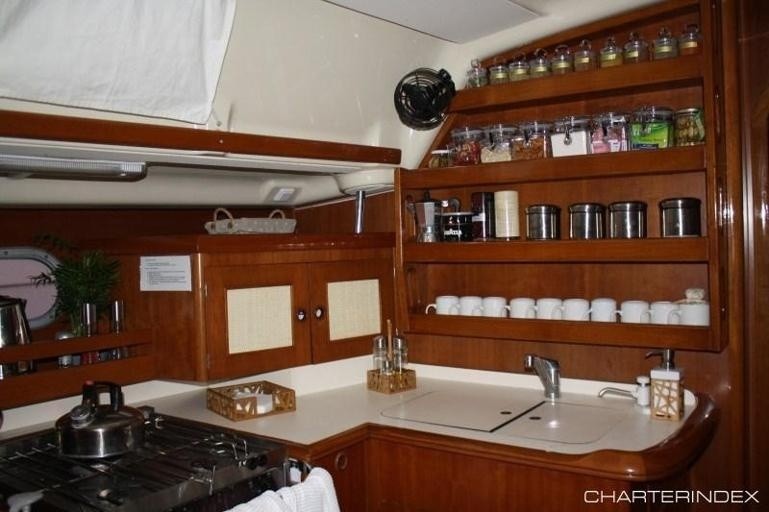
[0,405,294,512]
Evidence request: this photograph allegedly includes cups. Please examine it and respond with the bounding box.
[422,286,712,329]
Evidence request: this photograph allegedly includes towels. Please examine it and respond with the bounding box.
[275,465,341,511]
[223,487,296,511]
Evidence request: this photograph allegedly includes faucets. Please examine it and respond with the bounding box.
[597,375,651,407]
[524,353,561,398]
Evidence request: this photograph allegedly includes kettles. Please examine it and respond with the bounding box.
[0,295,35,381]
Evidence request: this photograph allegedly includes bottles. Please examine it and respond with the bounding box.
[53,329,75,369]
[472,188,523,242]
[83,303,101,363]
[524,205,561,243]
[464,22,701,92]
[354,191,367,233]
[111,299,126,359]
[428,103,703,170]
[391,335,409,386]
[658,197,702,239]
[373,333,387,374]
[607,200,647,239]
[567,200,606,239]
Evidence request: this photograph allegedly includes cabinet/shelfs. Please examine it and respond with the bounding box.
[303,439,697,511]
[389,51,729,356]
[0,310,154,419]
[60,227,396,388]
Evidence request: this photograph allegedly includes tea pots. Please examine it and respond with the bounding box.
[51,381,145,458]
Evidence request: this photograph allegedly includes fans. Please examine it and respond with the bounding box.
[392,66,458,133]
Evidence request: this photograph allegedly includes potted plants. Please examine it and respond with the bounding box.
[21,232,127,336]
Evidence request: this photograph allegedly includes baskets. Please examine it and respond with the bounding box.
[206,380,296,421]
